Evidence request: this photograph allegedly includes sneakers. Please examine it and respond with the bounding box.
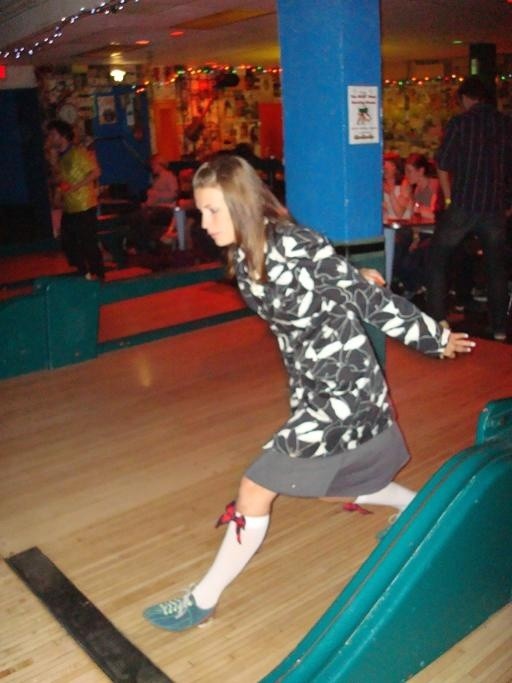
[143,594,216,632]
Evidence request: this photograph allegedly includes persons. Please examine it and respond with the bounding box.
[43,119,179,278]
[383,75,512,340]
[140,154,476,631]
